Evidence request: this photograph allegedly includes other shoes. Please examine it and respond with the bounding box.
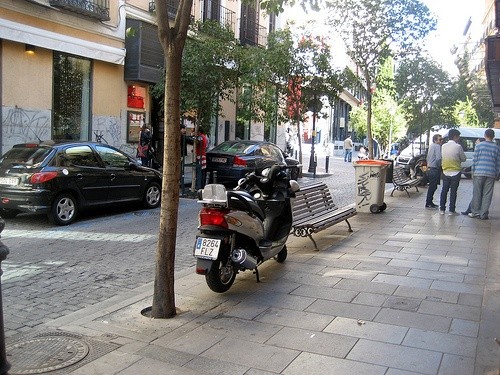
[431,202,439,208]
[448,211,460,215]
[425,205,436,209]
[344,160,347,162]
[460,211,468,215]
[348,160,352,162]
[189,187,199,192]
[439,209,445,214]
[468,211,480,218]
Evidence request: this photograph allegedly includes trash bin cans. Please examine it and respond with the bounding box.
[352,159,392,214]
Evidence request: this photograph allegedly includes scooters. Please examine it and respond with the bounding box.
[192,163,304,293]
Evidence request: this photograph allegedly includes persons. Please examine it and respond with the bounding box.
[425,134,442,210]
[179,124,209,192]
[136,127,152,169]
[344,135,353,163]
[461,128,500,221]
[441,129,466,217]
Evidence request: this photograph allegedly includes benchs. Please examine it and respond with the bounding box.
[390,167,421,198]
[288,182,358,251]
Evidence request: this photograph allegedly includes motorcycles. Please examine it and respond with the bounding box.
[408,155,429,187]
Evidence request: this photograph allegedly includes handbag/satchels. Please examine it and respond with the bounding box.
[136,144,149,158]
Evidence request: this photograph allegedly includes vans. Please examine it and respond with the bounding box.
[392,125,500,181]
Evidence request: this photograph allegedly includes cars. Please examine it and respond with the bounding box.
[0,124,164,225]
[195,137,301,194]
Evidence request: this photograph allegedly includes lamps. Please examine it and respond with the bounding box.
[25,44,35,54]
